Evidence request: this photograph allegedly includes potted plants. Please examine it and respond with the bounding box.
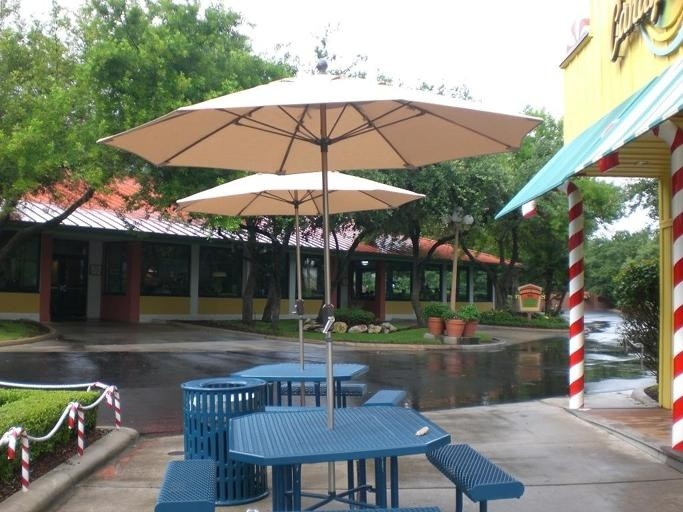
[441,308,472,337]
[457,304,482,337]
[423,299,448,336]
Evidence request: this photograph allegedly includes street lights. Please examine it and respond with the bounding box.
[440,205,474,311]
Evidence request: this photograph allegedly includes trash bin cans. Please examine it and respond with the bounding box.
[181,378,269,506]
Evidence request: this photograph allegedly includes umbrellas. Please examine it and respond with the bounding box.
[96,60,544,496]
[176,170,427,407]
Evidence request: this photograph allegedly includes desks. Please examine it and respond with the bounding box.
[229,362,369,408]
